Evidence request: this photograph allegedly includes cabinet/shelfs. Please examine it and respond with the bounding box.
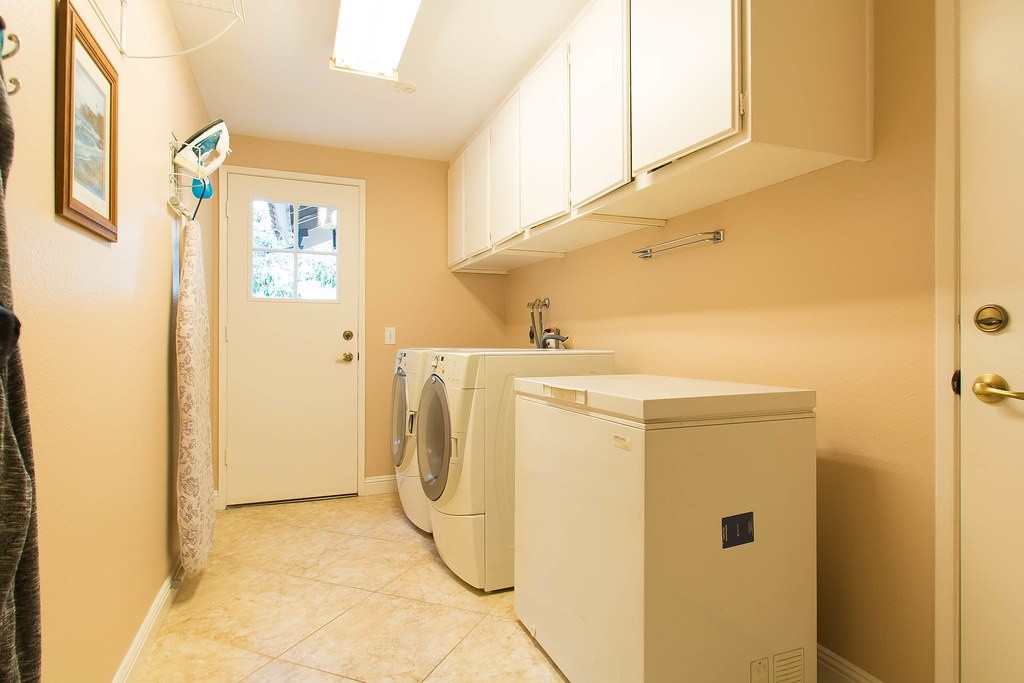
[446,0,874,275]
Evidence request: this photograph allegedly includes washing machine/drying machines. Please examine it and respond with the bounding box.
[393,348,561,534]
[416,350,614,593]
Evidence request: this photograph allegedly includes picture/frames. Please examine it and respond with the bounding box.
[56,0,118,242]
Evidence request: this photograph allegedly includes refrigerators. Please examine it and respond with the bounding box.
[512,374,817,683]
